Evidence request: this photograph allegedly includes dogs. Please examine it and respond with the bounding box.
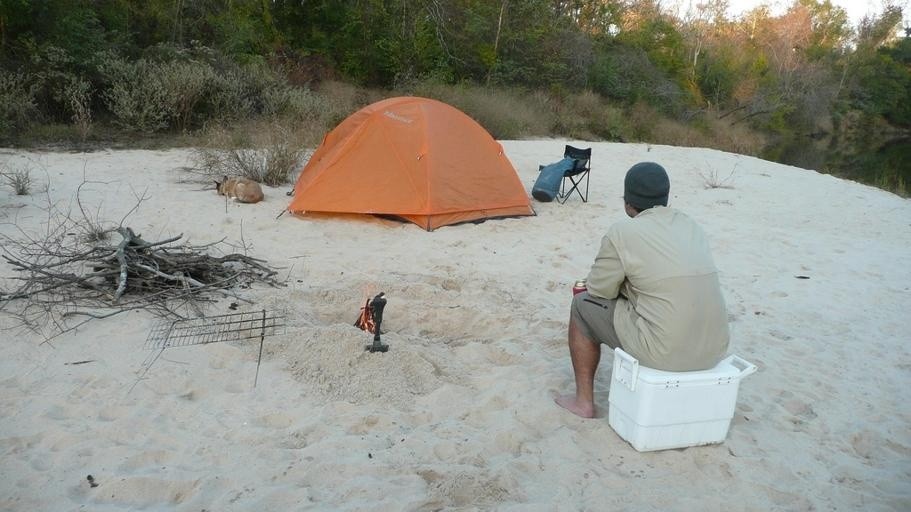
[213,174,264,204]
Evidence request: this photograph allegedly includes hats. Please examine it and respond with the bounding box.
[624,162,670,210]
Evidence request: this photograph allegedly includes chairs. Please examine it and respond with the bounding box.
[540,145,593,204]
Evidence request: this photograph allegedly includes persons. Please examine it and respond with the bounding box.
[553,162,730,419]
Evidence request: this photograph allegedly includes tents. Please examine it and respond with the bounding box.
[285,96,537,232]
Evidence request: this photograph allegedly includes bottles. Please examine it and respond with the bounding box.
[573,281,586,295]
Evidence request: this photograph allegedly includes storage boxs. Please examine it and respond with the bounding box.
[608,347,758,452]
